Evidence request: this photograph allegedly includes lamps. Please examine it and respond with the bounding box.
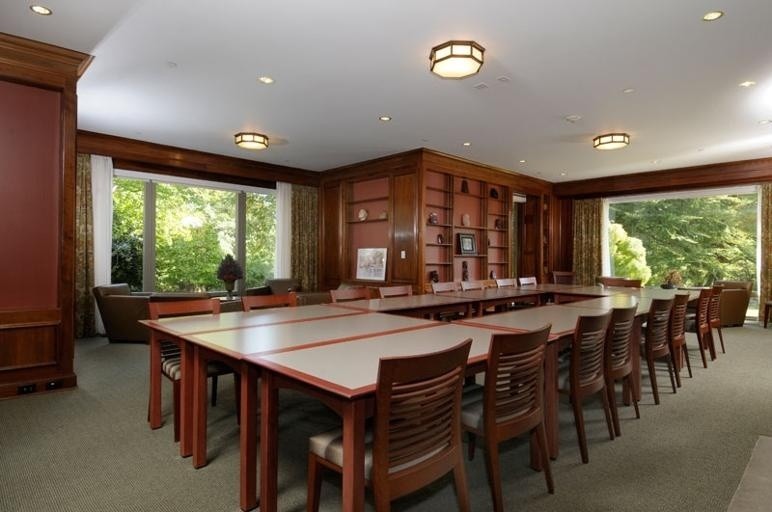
[593,133,631,151]
[429,40,485,80]
[234,133,270,151]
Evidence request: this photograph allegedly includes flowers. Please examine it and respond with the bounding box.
[215,254,244,283]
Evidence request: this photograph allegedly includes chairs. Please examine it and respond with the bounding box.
[763,288,772,328]
[92,282,156,345]
[713,279,754,328]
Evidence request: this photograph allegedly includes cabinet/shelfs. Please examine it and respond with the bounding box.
[522,193,553,305]
[424,169,512,319]
[346,194,390,225]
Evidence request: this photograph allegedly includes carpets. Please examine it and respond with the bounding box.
[727,432,772,512]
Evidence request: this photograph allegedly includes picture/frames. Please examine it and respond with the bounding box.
[355,246,389,283]
[458,232,478,257]
